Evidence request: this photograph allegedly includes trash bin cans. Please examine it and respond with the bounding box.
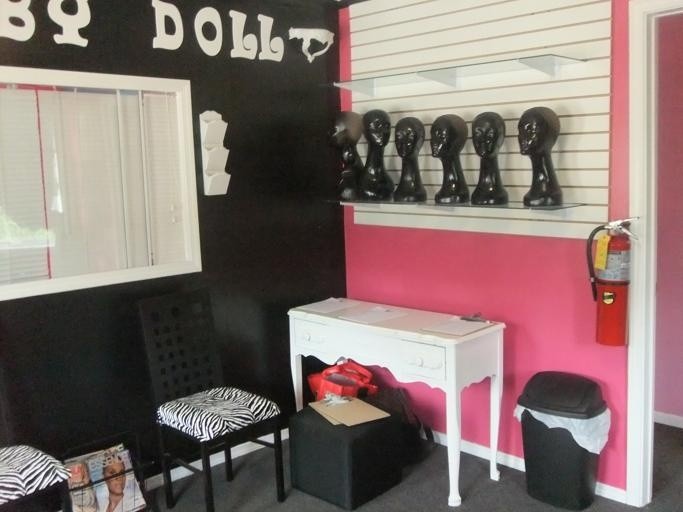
[516,370,607,512]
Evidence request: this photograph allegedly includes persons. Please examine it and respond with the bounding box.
[472,112,507,206]
[394,117,428,204]
[364,109,395,200]
[330,111,364,203]
[519,106,563,207]
[430,114,469,204]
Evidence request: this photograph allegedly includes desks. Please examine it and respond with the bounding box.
[287,297,505,507]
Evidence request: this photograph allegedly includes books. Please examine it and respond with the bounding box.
[64,443,145,512]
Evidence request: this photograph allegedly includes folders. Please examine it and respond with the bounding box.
[419,311,499,337]
[308,389,391,427]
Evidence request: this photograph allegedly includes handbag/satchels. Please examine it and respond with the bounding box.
[359,383,436,462]
[307,361,379,401]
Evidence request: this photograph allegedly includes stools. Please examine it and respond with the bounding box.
[291,396,402,512]
[137,287,284,511]
[0,446,71,512]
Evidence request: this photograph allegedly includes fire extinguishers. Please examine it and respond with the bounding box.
[587,218,639,346]
